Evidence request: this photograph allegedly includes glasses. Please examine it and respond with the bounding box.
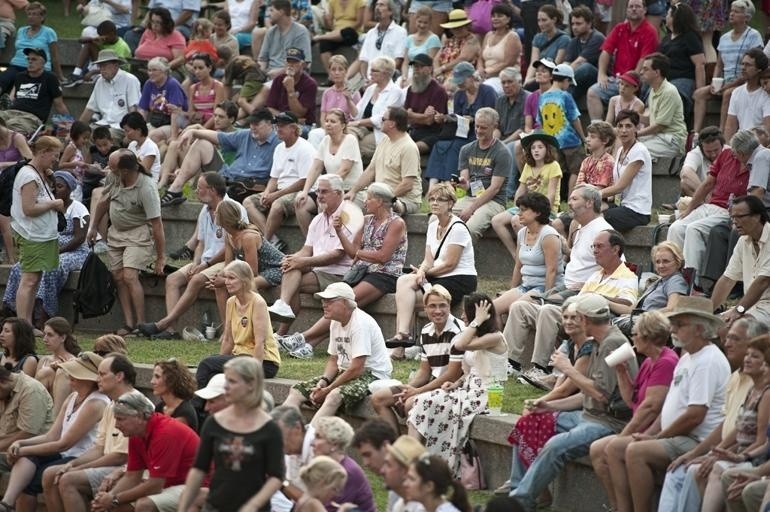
[730,214,751,220]
[75,353,96,368]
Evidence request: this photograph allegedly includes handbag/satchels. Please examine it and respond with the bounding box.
[458,441,487,492]
[57,210,66,233]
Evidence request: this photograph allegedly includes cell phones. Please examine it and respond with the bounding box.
[451,174,460,184]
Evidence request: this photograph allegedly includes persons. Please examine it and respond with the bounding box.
[0,0,770,511]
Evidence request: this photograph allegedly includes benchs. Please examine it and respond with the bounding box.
[0,32,726,511]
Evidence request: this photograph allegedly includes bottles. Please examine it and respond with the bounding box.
[728,193,736,209]
[553,339,570,378]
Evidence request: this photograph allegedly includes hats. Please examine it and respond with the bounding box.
[383,433,425,469]
[568,296,611,317]
[59,349,104,384]
[23,9,642,197]
[666,296,726,328]
[192,372,226,399]
[314,282,356,299]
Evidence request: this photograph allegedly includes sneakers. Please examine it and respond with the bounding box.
[166,246,193,269]
[109,299,414,360]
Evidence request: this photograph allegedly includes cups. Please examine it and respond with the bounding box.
[657,215,671,225]
[604,342,636,368]
[485,386,505,416]
[206,327,216,339]
[710,78,724,94]
